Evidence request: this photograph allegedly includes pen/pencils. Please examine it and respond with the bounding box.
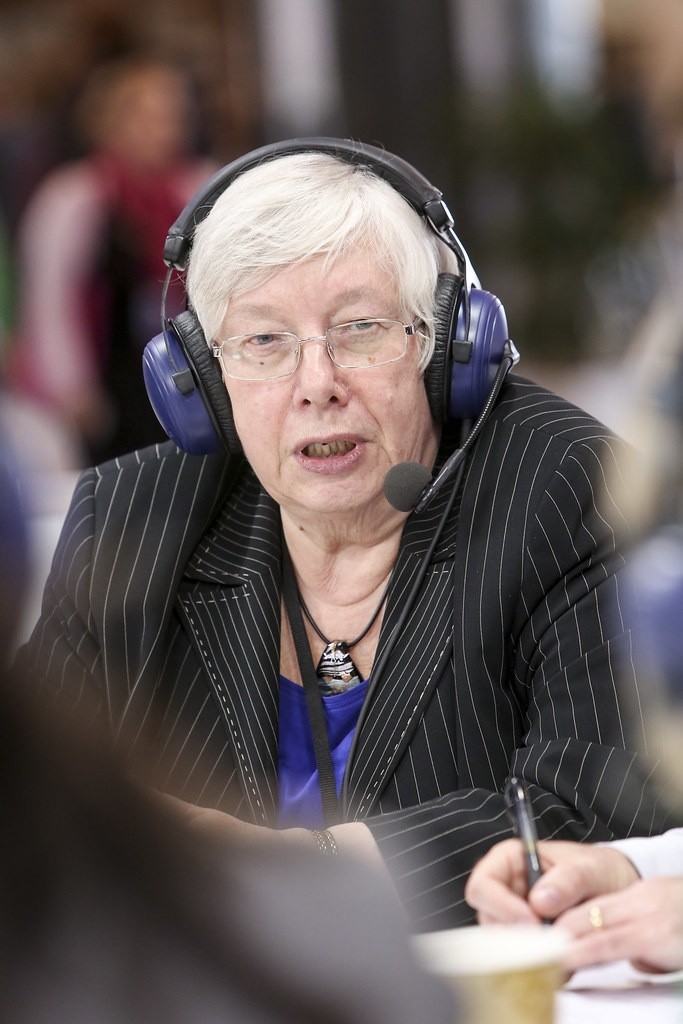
[505,773,544,885]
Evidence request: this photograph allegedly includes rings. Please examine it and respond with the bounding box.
[590,908,602,928]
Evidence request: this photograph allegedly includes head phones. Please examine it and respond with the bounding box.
[139,135,520,462]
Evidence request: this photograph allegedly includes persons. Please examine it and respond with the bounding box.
[465,828,683,986]
[0,143,683,944]
[11,53,233,466]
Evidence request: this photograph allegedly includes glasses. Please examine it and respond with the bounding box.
[207,316,423,381]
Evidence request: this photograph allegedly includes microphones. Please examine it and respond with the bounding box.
[380,357,509,516]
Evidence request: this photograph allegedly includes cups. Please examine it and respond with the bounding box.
[419,926,571,1024]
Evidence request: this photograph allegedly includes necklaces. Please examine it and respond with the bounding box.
[293,573,391,697]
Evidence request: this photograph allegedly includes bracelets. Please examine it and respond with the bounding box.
[310,828,337,855]
